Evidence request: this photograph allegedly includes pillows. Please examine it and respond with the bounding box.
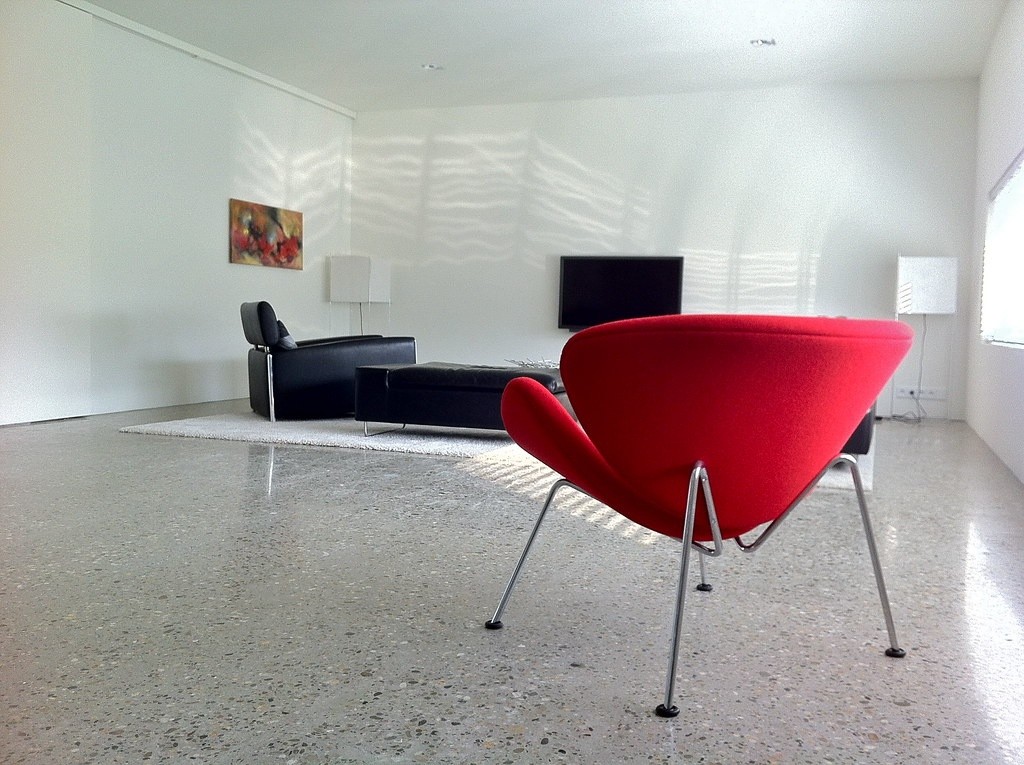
[276,319,298,348]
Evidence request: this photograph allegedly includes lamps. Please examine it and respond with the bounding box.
[329,256,393,335]
[894,254,960,425]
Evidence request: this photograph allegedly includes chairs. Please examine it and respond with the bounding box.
[482,311,917,717]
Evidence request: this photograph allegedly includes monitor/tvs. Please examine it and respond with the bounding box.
[557,256,684,329]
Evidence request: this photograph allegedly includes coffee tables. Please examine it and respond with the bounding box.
[355,361,559,437]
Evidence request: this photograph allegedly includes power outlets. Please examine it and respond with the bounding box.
[897,386,945,400]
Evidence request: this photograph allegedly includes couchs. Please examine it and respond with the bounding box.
[240,300,417,426]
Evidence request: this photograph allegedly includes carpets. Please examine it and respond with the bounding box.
[119,416,876,491]
